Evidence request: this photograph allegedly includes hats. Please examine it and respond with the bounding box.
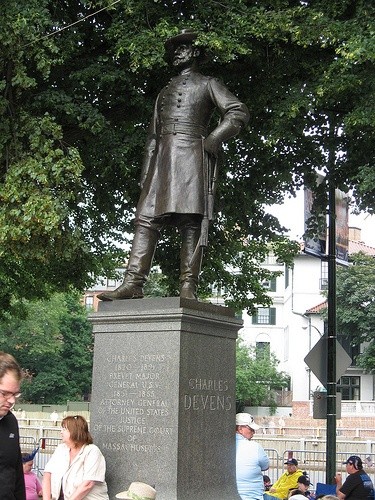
[115,482,157,500]
[298,476,313,485]
[342,456,362,470]
[284,458,298,465]
[236,413,260,430]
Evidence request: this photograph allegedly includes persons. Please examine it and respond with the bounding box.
[21,452,44,500]
[115,482,157,500]
[236,413,270,500]
[335,455,375,500]
[288,474,316,500]
[0,350,26,500]
[95,31,252,303]
[288,489,310,500]
[263,475,271,491]
[42,415,109,500]
[263,458,303,500]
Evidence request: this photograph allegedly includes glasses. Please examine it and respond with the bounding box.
[0,390,23,399]
